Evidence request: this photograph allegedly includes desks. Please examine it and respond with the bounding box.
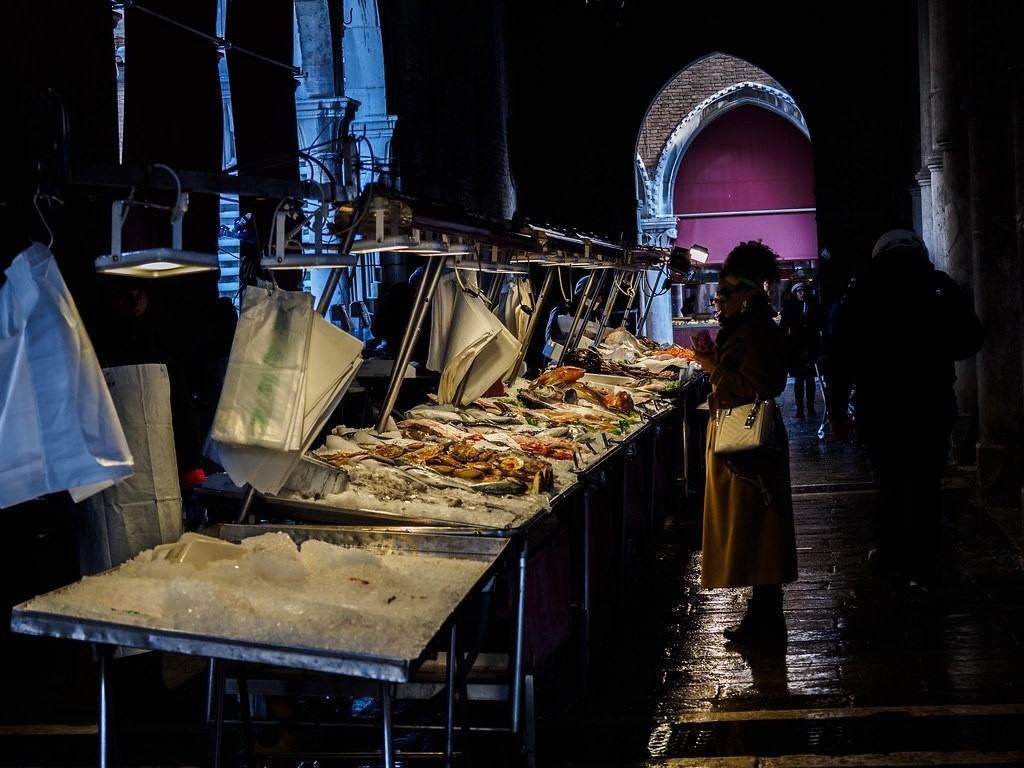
[9,371,707,767]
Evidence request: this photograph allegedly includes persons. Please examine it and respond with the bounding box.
[529,273,603,380]
[690,215,987,645]
[370,266,440,423]
[81,269,237,452]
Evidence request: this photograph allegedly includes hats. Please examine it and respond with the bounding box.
[872,230,929,259]
[791,280,808,294]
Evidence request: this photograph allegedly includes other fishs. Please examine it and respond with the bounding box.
[395,366,691,461]
[318,449,554,508]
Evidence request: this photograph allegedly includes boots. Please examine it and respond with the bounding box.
[806,401,816,417]
[723,598,787,642]
[795,402,805,418]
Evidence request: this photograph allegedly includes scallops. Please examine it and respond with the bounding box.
[355,430,553,482]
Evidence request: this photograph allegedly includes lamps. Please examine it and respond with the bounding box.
[688,243,709,283]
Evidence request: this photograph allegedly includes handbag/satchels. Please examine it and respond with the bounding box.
[714,388,775,457]
